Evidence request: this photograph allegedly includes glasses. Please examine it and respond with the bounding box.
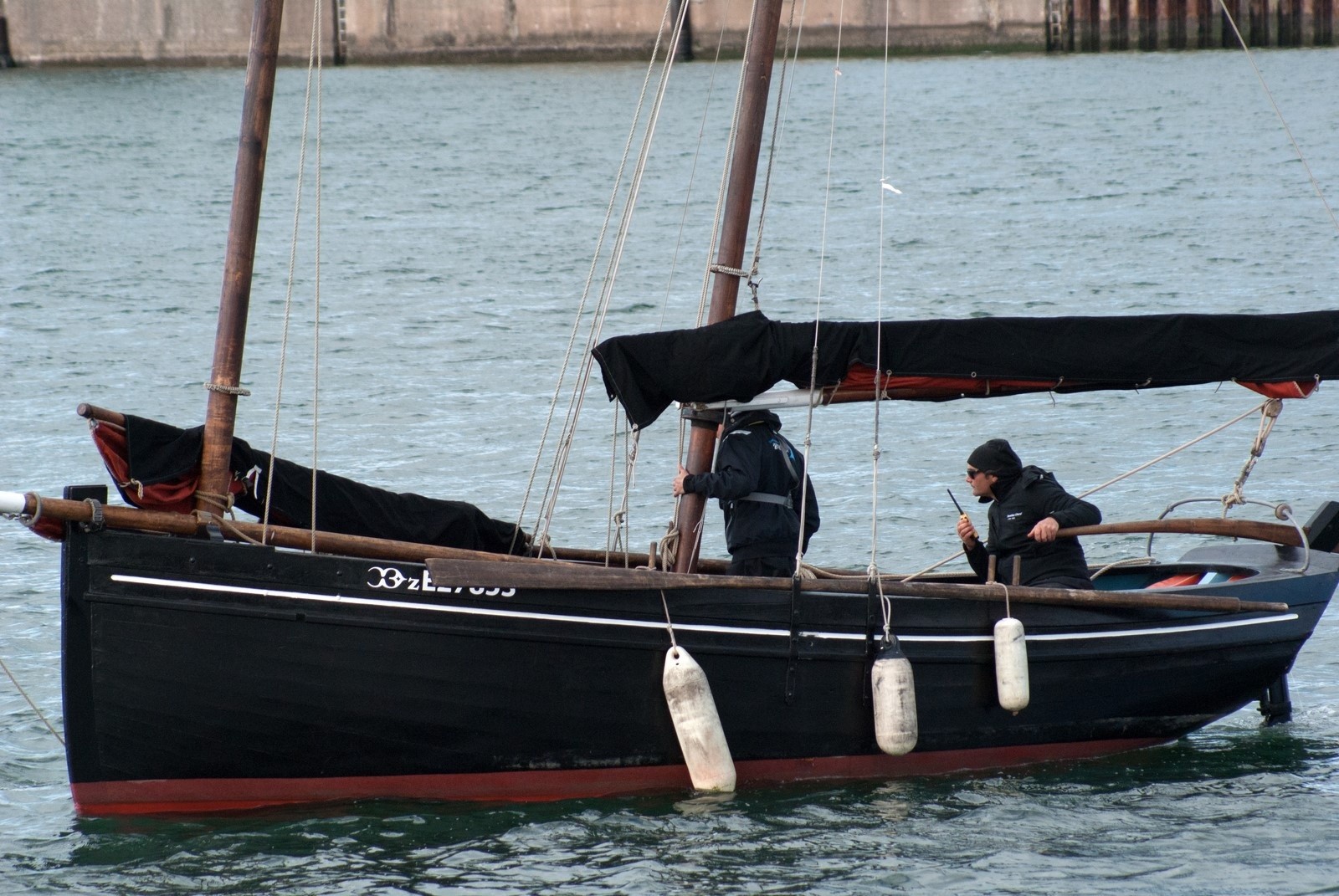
[966,469,981,480]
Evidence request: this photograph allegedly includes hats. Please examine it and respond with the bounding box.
[967,439,1022,482]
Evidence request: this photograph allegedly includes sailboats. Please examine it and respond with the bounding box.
[0,0,1336,811]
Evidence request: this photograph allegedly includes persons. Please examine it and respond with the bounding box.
[674,406,821,577]
[956,437,1102,590]
